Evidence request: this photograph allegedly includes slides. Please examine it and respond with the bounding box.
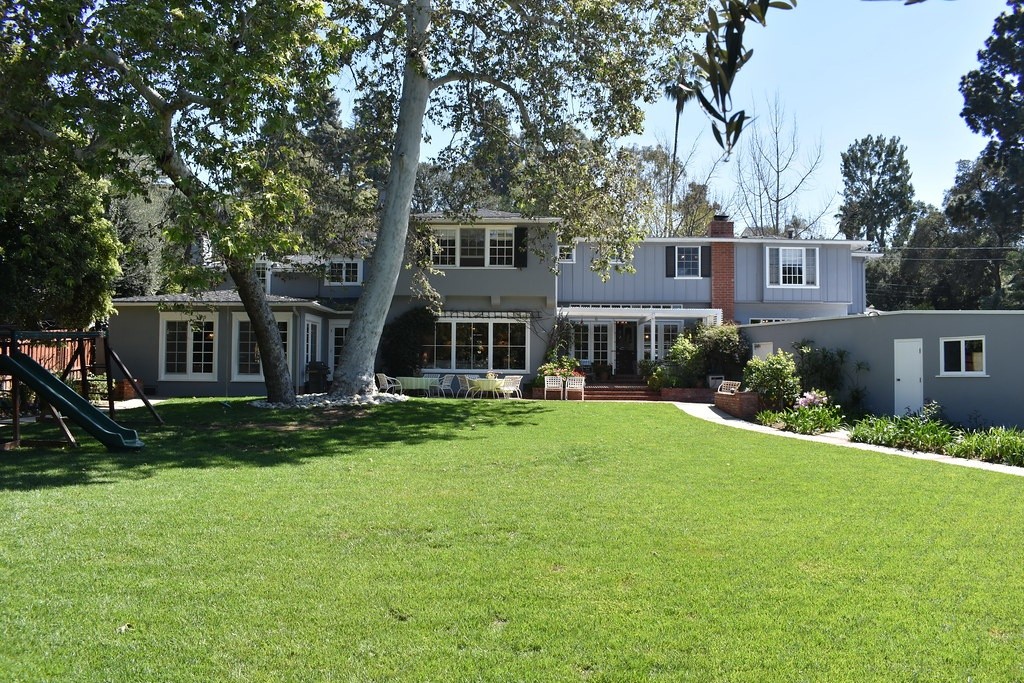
[0,352,146,452]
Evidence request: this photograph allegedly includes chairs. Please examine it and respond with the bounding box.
[544,375,564,400]
[565,377,585,401]
[423,374,522,399]
[717,380,741,394]
[375,374,403,396]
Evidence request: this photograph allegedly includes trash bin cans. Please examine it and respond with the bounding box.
[306,361,332,393]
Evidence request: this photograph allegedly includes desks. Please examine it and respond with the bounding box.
[467,378,505,399]
[395,376,438,397]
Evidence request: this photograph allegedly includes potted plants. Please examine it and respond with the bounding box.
[597,366,612,380]
[664,319,751,389]
